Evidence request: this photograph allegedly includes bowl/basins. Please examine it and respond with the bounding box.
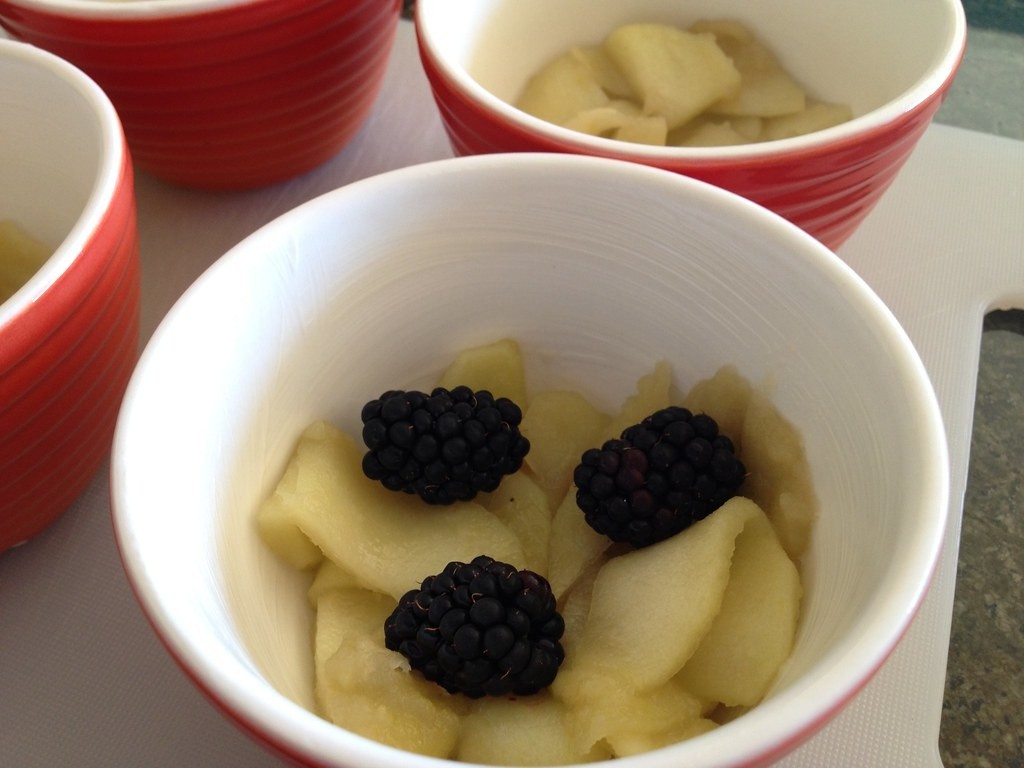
[0,37,143,557]
[413,0,967,251]
[1,0,402,194]
[106,150,949,768]
